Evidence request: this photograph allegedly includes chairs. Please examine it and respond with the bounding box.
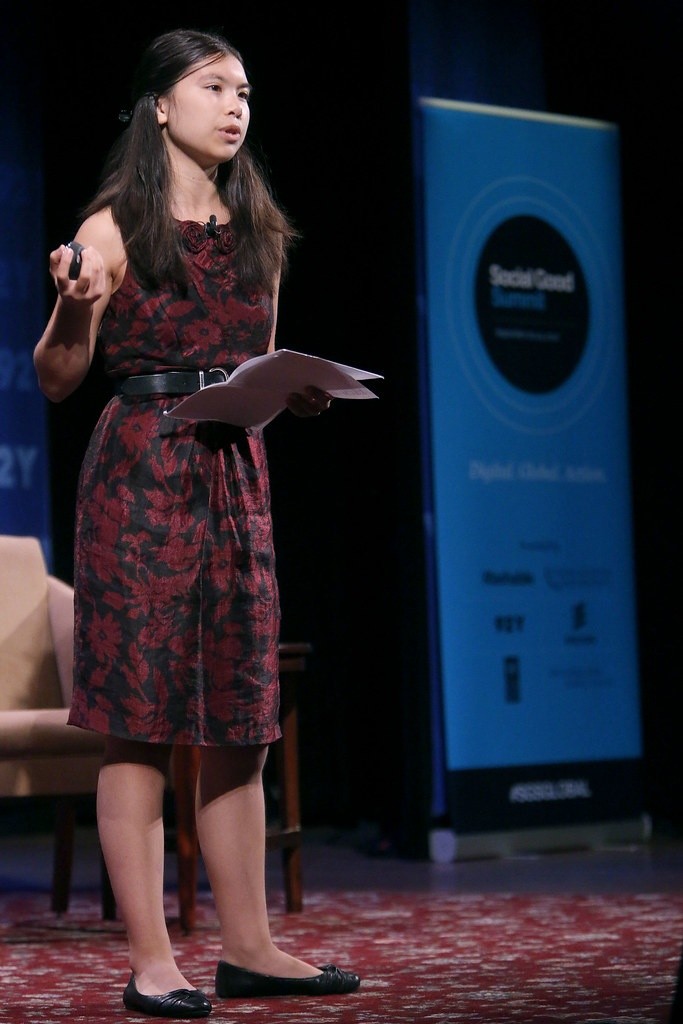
[0,535,172,923]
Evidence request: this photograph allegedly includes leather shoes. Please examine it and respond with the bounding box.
[122,974,212,1017]
[215,960,360,999]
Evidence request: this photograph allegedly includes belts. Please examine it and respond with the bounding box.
[120,367,229,396]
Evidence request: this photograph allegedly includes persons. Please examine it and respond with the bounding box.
[33,30,362,1019]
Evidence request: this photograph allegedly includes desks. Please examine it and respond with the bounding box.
[173,644,314,937]
